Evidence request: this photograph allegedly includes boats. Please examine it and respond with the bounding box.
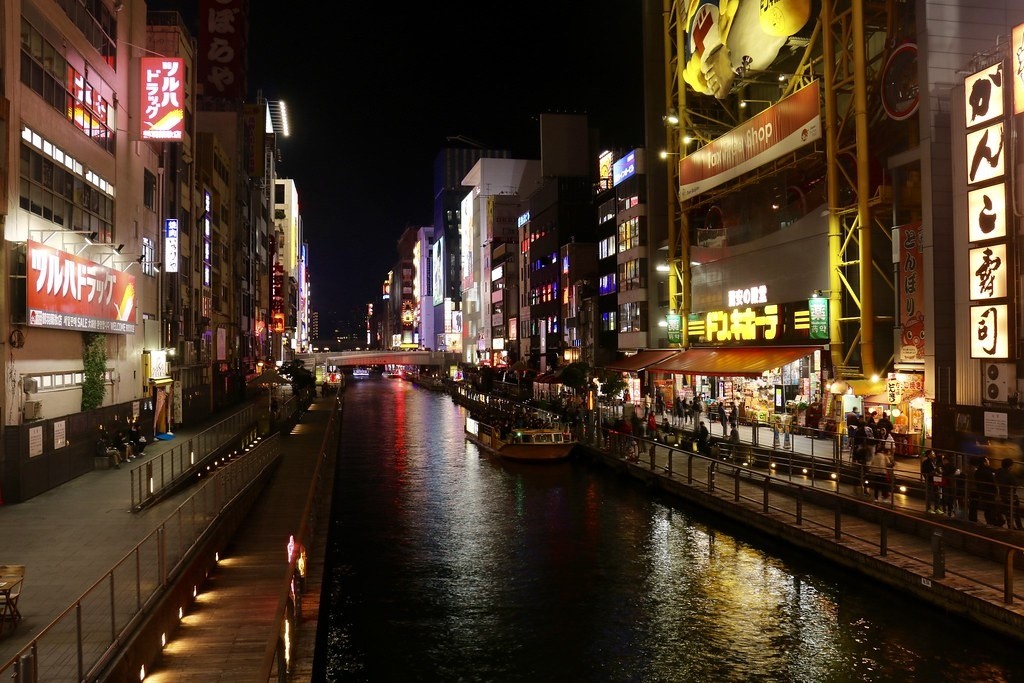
[464,416,579,462]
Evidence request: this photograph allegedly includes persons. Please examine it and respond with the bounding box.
[419,375,741,467]
[599,166,614,191]
[846,407,1024,531]
[96,422,149,469]
[267,397,278,413]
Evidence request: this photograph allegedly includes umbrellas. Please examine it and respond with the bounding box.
[248,368,290,415]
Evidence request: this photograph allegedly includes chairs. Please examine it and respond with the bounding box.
[0,565,25,616]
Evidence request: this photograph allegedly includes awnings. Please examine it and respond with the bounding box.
[533,374,562,384]
[604,346,823,377]
[477,359,526,373]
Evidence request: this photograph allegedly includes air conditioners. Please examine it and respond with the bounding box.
[984,362,1015,403]
[24,377,38,393]
[24,400,42,420]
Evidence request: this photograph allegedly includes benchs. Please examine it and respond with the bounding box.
[94,450,124,469]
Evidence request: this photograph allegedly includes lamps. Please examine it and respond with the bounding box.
[811,289,831,297]
[657,56,820,159]
[30,229,162,274]
[160,340,176,355]
[269,99,290,138]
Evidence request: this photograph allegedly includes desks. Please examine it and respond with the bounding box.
[0,577,24,622]
[892,431,922,455]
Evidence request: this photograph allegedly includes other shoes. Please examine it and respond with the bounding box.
[864,492,869,496]
[139,453,145,455]
[115,464,120,469]
[936,509,944,514]
[124,459,131,462]
[129,455,136,459]
[928,509,935,514]
